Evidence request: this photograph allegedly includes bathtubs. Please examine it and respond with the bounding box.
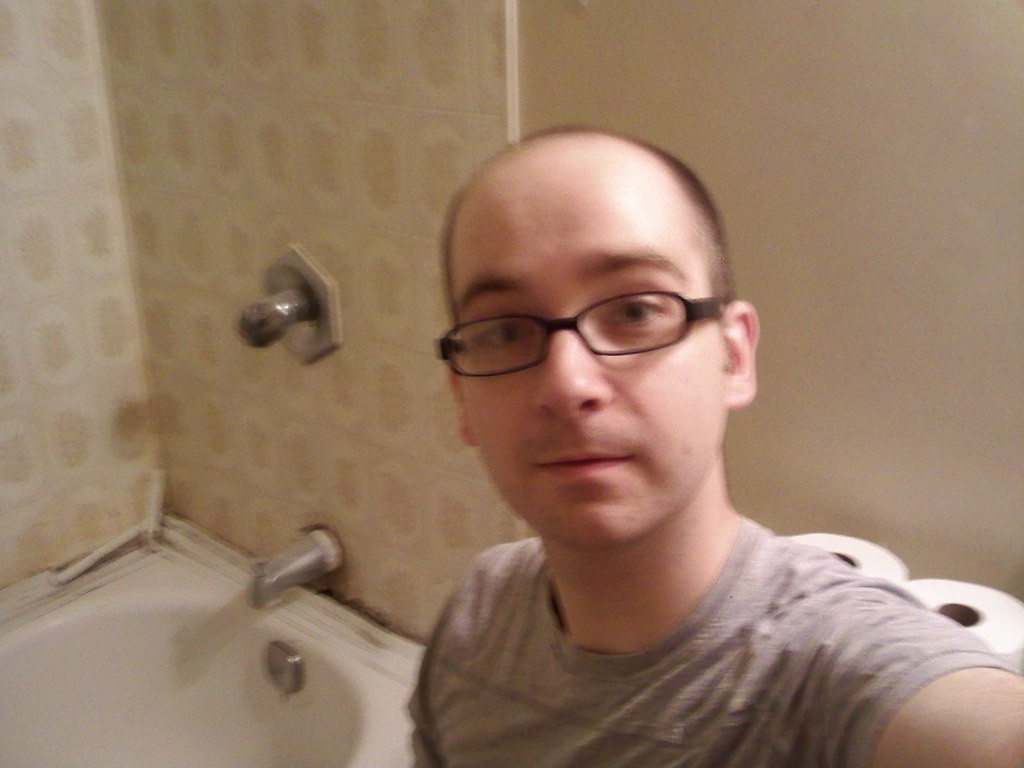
[2,515,427,766]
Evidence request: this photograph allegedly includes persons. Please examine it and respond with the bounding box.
[413,126,1024,768]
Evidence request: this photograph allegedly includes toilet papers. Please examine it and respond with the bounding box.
[902,577,1023,680]
[789,534,909,584]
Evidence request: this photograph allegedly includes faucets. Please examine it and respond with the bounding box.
[245,527,346,610]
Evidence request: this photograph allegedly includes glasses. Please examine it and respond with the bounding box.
[435,290,725,377]
